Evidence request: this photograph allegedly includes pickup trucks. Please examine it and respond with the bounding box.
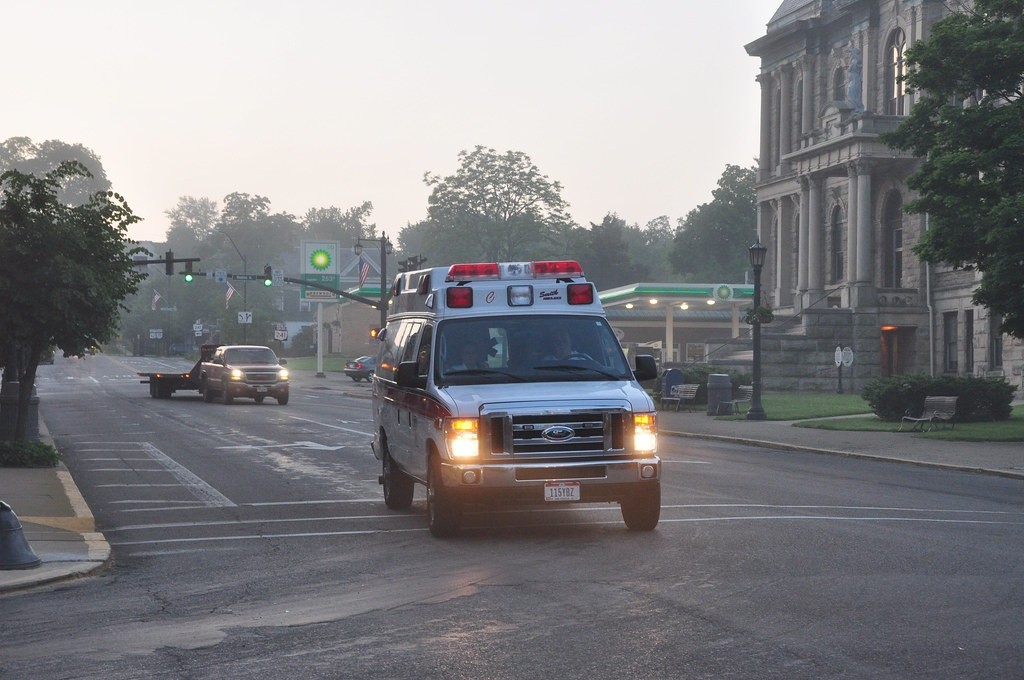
[199,346,291,406]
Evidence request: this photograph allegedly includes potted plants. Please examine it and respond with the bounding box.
[740,306,774,325]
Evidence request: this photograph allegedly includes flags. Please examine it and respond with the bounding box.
[359,254,370,288]
[225,281,235,309]
[152,289,161,311]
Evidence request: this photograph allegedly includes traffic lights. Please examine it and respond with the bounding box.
[370,327,379,340]
[397,260,407,272]
[184,261,194,282]
[264,265,272,288]
[406,256,418,270]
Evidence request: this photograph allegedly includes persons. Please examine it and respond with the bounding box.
[444,342,489,372]
[542,325,593,361]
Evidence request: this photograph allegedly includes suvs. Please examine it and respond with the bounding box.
[367,259,664,542]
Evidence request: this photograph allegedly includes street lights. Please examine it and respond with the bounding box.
[354,229,389,343]
[744,240,769,420]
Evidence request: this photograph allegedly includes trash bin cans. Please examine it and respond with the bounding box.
[662,369,683,401]
[706,374,733,415]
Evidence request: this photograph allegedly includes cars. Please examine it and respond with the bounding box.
[345,355,376,383]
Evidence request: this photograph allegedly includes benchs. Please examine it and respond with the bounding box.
[661,383,700,413]
[899,396,959,433]
[716,385,753,415]
